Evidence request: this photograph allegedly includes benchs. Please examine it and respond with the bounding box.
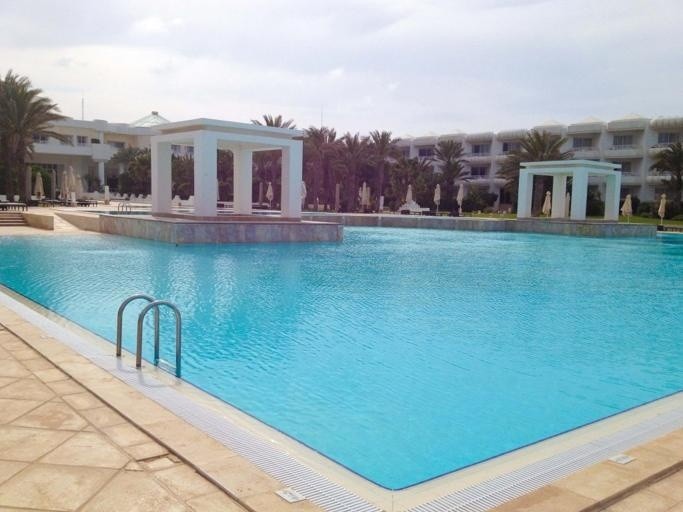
[657,225,683,231]
[401,209,466,216]
[0,199,98,212]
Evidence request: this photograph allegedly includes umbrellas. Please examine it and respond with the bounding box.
[542,190,551,217]
[266,182,273,208]
[405,184,413,214]
[657,193,667,224]
[456,182,464,215]
[34,171,43,200]
[433,183,441,212]
[360,182,370,212]
[620,193,632,224]
[301,181,306,210]
[60,164,82,205]
